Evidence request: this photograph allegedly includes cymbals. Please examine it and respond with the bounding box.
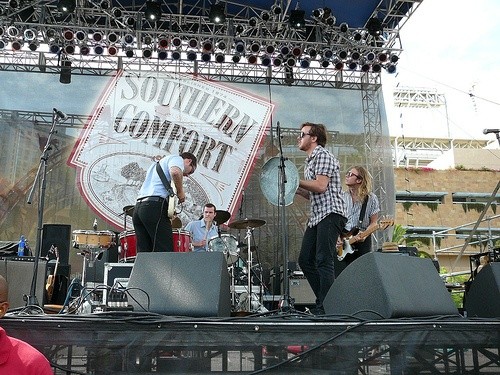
[198,210,230,226]
[171,217,182,229]
[123,205,135,217]
[228,219,266,229]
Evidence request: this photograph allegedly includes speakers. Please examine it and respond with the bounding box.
[125,252,232,319]
[83,248,118,286]
[280,279,317,304]
[464,262,500,320]
[323,251,459,320]
[0,259,47,312]
[47,264,71,306]
[41,225,71,264]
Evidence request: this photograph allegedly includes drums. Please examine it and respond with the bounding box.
[171,228,195,253]
[119,229,137,263]
[207,235,240,267]
[72,229,116,248]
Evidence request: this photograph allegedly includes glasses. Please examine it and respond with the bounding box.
[300,132,314,138]
[347,172,362,180]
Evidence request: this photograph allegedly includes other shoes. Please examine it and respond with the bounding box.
[310,306,325,316]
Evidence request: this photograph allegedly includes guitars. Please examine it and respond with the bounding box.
[168,180,175,217]
[45,247,60,301]
[336,217,394,262]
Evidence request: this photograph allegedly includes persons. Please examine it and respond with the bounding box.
[295,122,349,315]
[334,166,380,280]
[185,203,219,252]
[0,275,54,375]
[132,152,197,253]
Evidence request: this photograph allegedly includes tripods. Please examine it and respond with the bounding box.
[0,112,60,316]
[231,139,314,316]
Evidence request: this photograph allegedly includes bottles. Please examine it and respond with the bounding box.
[17,235,29,256]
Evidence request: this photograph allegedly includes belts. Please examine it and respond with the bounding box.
[137,197,164,202]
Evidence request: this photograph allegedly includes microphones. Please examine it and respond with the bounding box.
[277,121,280,136]
[53,108,68,119]
[483,129,500,134]
